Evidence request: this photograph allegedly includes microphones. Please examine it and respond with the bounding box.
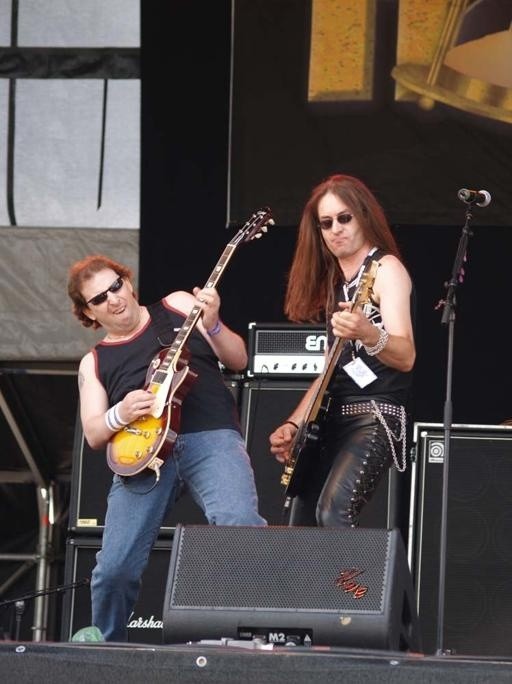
[457,185,493,213]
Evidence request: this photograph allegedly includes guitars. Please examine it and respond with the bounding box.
[277,250,380,498]
[106,204,275,478]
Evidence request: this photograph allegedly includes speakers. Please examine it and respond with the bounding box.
[61,539,173,646]
[239,376,400,529]
[161,521,424,654]
[415,430,512,662]
[69,382,243,532]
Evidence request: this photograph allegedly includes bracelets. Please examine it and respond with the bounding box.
[207,320,221,335]
[105,402,128,432]
[282,421,299,430]
[364,325,388,356]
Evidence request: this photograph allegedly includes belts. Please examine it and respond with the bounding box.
[327,402,407,420]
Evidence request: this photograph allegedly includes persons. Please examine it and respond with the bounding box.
[269,175,416,528]
[68,256,268,641]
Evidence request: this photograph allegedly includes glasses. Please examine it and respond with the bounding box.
[315,214,352,230]
[86,275,122,307]
